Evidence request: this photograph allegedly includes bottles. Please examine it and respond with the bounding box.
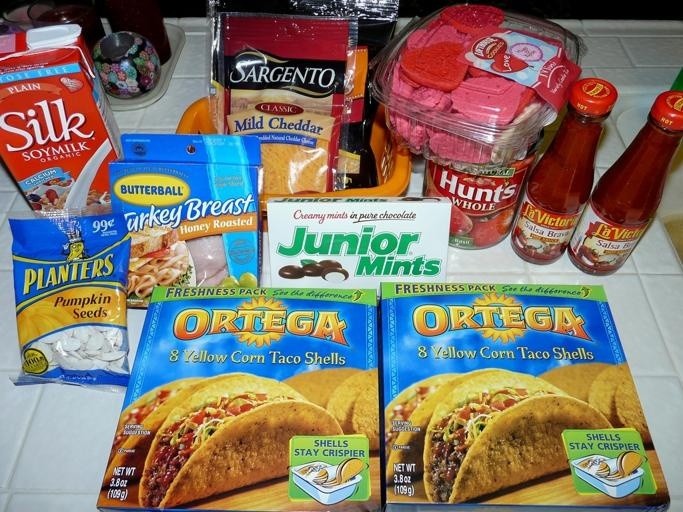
[566,90,682,277]
[510,76,619,265]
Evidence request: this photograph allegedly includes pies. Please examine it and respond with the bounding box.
[101,367,380,510]
[384,363,651,506]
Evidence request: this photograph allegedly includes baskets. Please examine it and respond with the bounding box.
[173,94,412,232]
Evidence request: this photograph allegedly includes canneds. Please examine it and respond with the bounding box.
[423,130,545,251]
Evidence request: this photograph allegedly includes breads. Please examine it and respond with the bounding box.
[388,4,571,165]
